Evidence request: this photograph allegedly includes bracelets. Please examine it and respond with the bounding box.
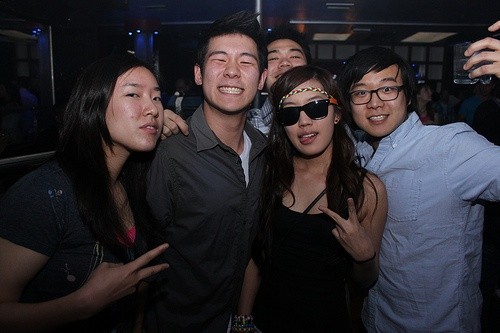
[232,315,257,333]
[353,252,376,264]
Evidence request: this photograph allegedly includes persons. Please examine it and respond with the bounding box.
[0,9,500,333]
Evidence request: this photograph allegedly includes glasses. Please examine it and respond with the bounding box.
[276,98,339,126]
[347,84,406,105]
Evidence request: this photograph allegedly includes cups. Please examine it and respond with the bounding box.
[453,44,491,85]
